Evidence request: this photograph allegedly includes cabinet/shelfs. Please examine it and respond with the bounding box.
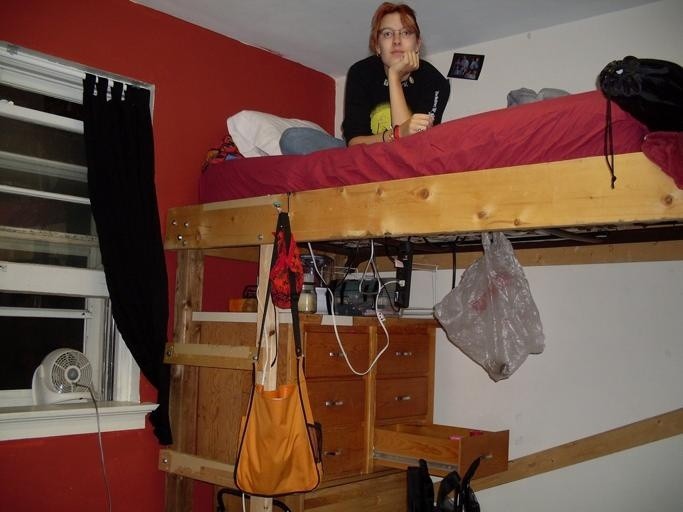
[197,310,509,512]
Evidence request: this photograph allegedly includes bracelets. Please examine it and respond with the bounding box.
[382,125,400,143]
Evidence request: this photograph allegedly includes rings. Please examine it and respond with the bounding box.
[417,129,421,132]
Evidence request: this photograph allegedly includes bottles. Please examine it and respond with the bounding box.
[296,274,317,314]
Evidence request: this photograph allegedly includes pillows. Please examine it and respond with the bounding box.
[226,110,335,158]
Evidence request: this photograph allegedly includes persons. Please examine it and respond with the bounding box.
[279,2,450,155]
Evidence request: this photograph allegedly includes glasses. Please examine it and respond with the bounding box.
[383,29,408,39]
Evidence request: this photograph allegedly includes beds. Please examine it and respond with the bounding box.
[161,87,682,511]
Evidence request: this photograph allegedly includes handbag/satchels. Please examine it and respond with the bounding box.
[235,356,323,497]
[600,56,683,134]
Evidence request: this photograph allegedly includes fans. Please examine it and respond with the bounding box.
[31,347,93,407]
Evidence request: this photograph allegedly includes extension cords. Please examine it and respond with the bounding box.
[394,255,413,307]
